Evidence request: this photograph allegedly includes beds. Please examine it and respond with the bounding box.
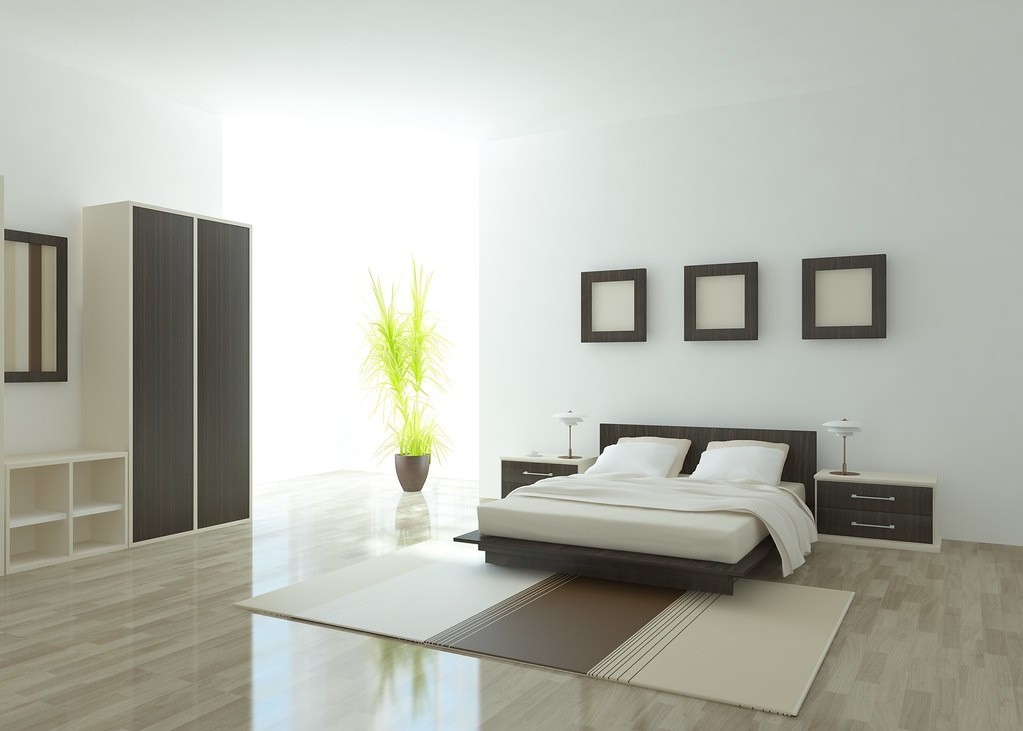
[452,419,820,594]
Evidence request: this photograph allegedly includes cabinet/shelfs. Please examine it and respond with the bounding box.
[82,196,256,546]
[6,449,128,577]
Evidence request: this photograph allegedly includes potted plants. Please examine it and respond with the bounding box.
[347,256,450,495]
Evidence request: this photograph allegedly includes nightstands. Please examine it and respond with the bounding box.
[811,470,946,552]
[493,450,599,500]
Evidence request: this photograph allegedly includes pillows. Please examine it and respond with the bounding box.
[689,445,785,485]
[706,438,790,486]
[616,436,693,478]
[584,441,679,478]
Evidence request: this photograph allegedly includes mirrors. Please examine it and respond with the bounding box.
[3,228,70,384]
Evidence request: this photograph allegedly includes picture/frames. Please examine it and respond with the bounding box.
[678,262,760,341]
[796,253,889,341]
[578,268,649,343]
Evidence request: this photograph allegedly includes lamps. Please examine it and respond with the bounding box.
[549,410,588,459]
[820,417,868,476]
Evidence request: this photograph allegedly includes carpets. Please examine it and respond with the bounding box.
[229,535,861,718]
